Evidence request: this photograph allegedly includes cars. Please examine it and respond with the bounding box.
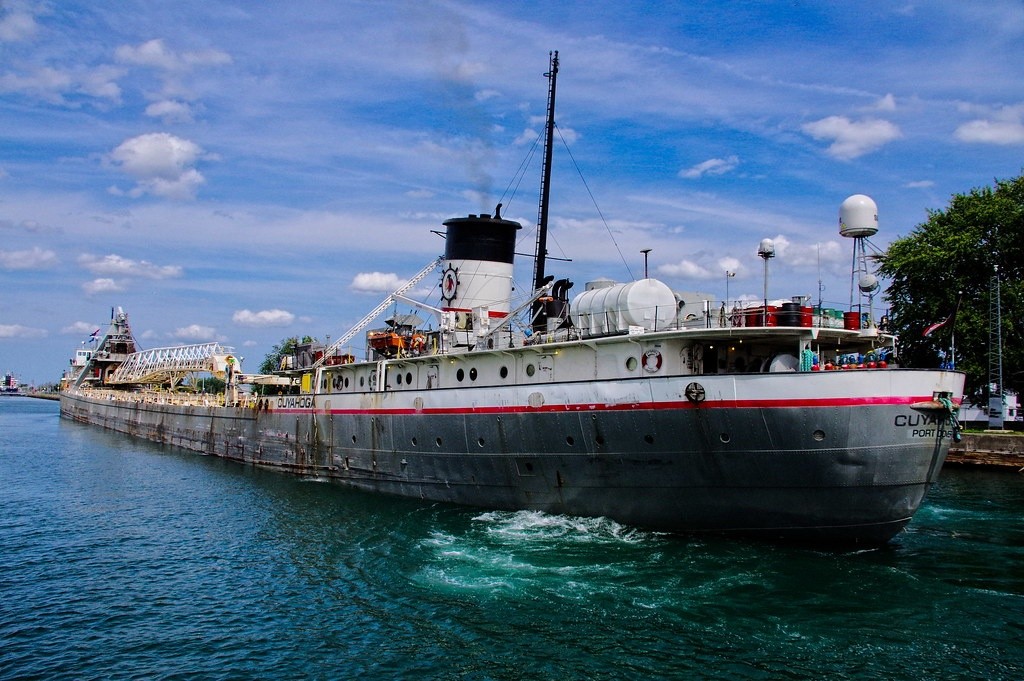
[1014,413,1023,422]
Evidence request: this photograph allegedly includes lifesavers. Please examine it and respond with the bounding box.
[641,350,662,372]
[411,337,423,355]
[336,376,343,390]
[731,306,744,327]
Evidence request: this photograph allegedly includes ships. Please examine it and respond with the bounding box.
[61,49,968,558]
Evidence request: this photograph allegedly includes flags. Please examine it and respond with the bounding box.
[89,329,100,336]
[922,298,958,337]
[88,337,94,342]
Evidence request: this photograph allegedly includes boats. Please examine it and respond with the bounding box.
[0,369,26,396]
